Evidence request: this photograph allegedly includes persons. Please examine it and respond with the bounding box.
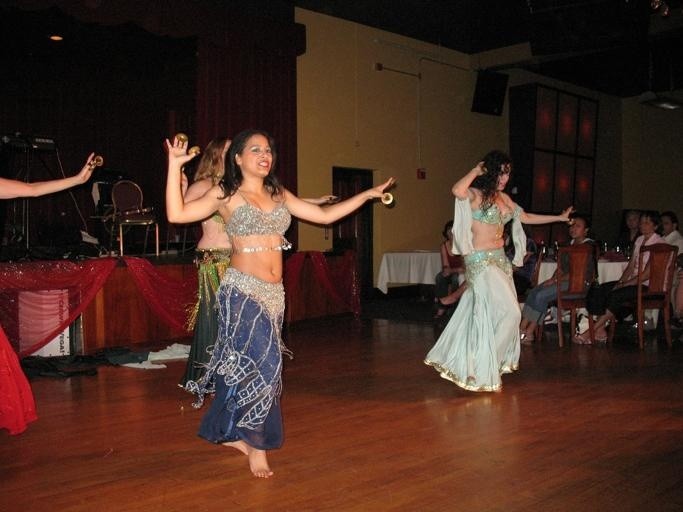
[0,152,98,437]
[165,126,398,478]
[164,130,338,398]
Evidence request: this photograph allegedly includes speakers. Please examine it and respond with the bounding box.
[471,69,509,117]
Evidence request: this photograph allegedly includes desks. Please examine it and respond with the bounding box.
[376,245,443,303]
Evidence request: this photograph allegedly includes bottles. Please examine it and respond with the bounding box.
[538,239,631,263]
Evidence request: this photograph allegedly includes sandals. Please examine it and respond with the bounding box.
[573,335,607,344]
[435,297,457,308]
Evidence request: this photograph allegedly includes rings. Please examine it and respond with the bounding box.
[188,146,201,155]
[94,155,103,166]
[382,193,394,205]
[176,132,188,142]
[384,175,395,181]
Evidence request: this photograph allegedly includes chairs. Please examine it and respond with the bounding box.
[500,235,679,356]
[108,175,161,256]
